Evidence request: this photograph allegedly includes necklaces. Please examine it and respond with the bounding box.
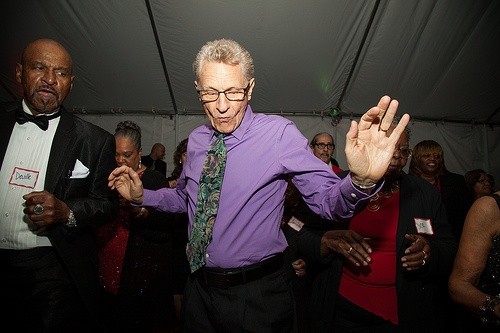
[367,181,400,212]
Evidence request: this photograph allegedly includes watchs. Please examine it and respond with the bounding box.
[66,209,77,227]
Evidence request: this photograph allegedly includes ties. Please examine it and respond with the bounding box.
[186,131,229,274]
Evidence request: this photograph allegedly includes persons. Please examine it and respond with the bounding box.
[0,38,119,333]
[86,121,189,333]
[107,38,410,333]
[277,114,500,333]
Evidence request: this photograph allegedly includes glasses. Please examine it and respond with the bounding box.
[477,179,490,184]
[394,146,413,159]
[196,83,250,104]
[315,143,335,150]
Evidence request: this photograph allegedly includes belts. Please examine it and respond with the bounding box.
[0,247,78,263]
[126,288,163,297]
[197,255,284,288]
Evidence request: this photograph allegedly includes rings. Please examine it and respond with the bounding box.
[34,204,43,215]
[347,247,352,253]
[379,127,390,132]
[422,250,427,259]
[422,260,425,265]
[295,270,300,275]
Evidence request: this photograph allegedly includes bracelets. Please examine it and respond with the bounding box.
[351,179,377,194]
[482,294,496,312]
[135,207,145,219]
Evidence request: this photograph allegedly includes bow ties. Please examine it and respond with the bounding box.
[14,108,64,131]
